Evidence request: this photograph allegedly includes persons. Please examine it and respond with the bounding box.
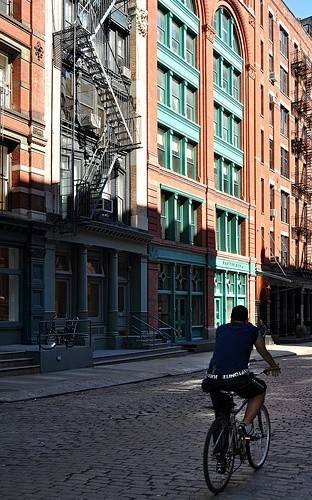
[257,319,267,342]
[205,305,281,473]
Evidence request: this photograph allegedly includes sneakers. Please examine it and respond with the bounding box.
[216,462,227,473]
[237,423,260,439]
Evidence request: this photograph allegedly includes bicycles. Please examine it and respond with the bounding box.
[203,362,282,494]
[36,314,79,350]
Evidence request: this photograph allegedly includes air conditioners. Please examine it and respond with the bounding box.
[80,112,101,128]
[121,65,133,78]
[130,214,149,230]
[270,71,278,82]
[95,196,114,214]
[269,94,277,105]
[271,208,277,217]
